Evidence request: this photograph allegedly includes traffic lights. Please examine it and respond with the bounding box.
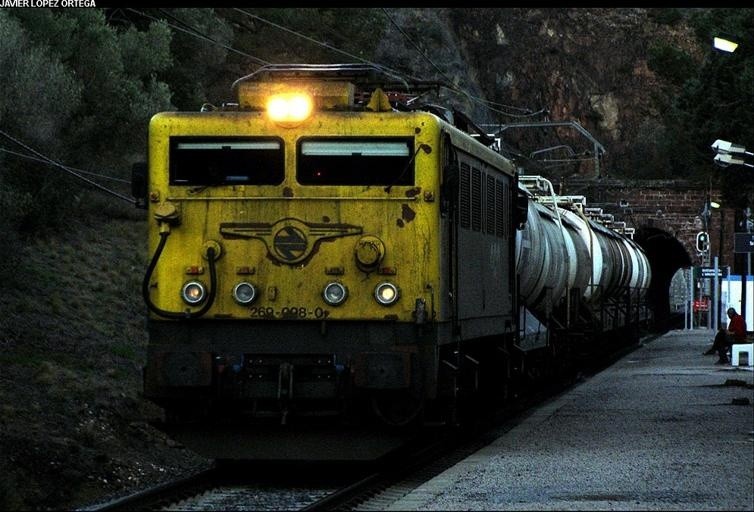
[696,231,710,253]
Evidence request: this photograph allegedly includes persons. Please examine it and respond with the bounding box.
[702,307,749,365]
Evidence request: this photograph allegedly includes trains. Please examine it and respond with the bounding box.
[144,44,660,468]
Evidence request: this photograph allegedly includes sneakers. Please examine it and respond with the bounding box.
[703,349,716,356]
[714,359,729,365]
